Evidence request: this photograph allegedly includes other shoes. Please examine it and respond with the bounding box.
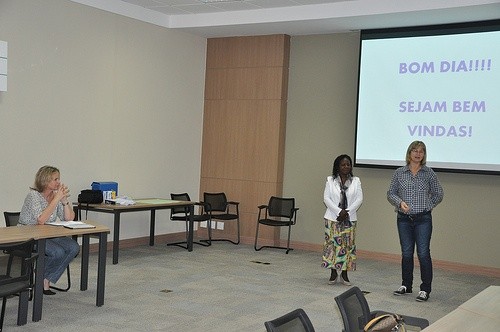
[43,288,57,295]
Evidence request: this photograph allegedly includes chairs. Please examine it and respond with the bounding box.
[265,309,314,332]
[167,192,240,249]
[0,212,71,332]
[334,286,429,332]
[254,196,299,254]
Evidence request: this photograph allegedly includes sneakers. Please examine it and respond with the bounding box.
[393,285,412,295]
[416,291,429,302]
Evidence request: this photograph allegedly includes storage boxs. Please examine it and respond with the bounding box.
[91,182,118,203]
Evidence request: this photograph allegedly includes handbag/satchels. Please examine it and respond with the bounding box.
[364,313,406,332]
[78,189,102,203]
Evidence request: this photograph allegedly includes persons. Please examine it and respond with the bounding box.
[323,154,363,285]
[16,165,80,295]
[387,141,444,302]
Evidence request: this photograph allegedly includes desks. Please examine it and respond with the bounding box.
[419,285,500,332]
[78,198,194,264]
[0,220,110,323]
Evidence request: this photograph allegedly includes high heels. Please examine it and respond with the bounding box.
[328,274,338,284]
[340,274,351,286]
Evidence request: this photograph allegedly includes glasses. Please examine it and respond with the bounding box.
[411,150,423,153]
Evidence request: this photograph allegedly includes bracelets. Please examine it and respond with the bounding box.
[62,201,69,206]
